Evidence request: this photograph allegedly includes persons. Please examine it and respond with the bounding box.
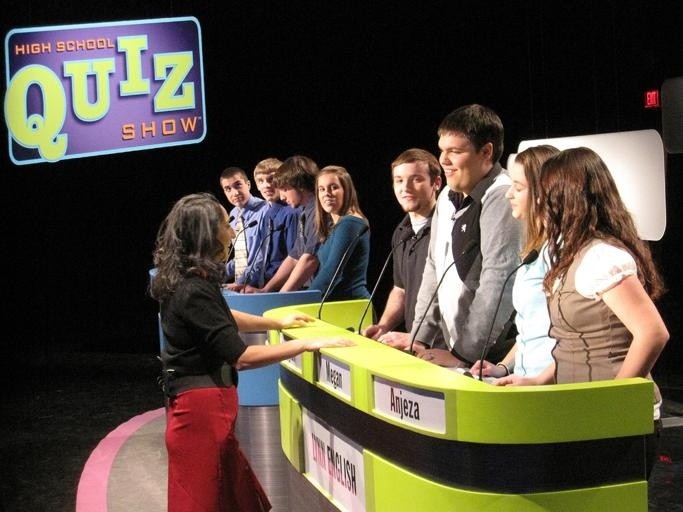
[537,147,670,481]
[470,145,561,386]
[405,105,526,369]
[355,148,449,352]
[157,191,353,512]
[219,156,377,325]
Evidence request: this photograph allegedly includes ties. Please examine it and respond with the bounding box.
[234,216,248,282]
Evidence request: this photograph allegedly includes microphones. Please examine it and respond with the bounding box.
[403,239,477,355]
[225,220,257,261]
[318,225,369,321]
[358,231,409,335]
[477,249,538,381]
[242,223,285,293]
[228,216,235,224]
